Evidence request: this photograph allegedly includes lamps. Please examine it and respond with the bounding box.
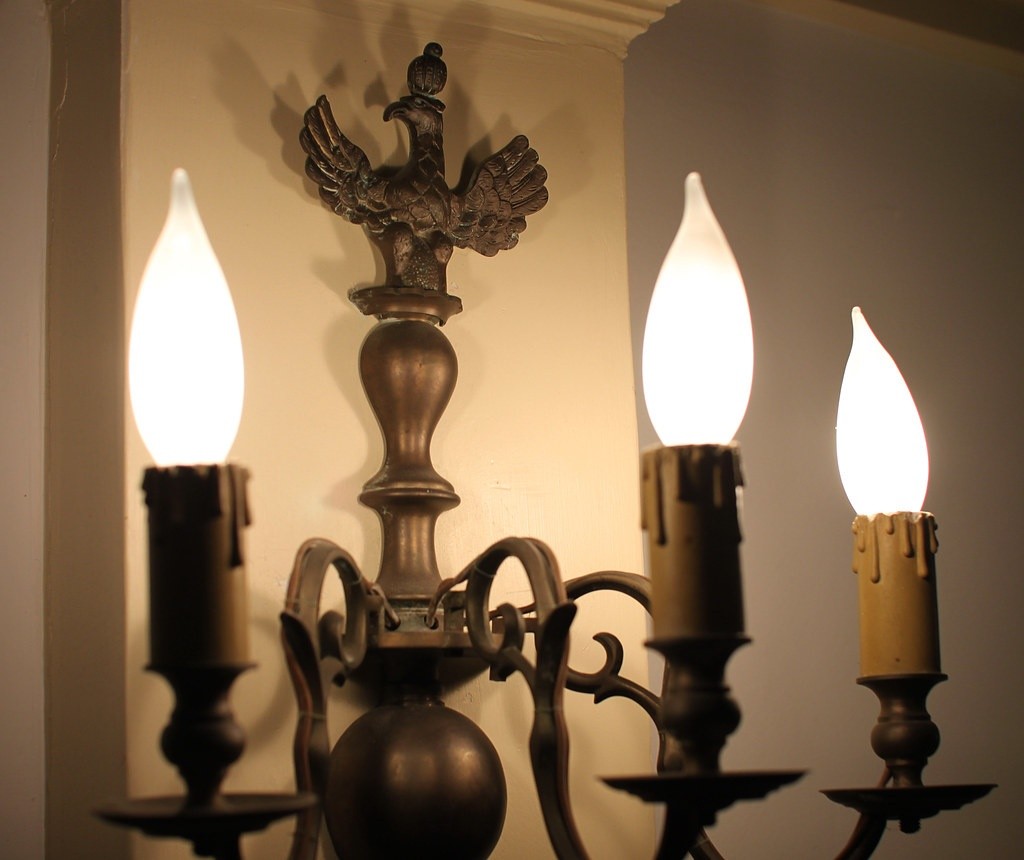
[87,39,1004,859]
